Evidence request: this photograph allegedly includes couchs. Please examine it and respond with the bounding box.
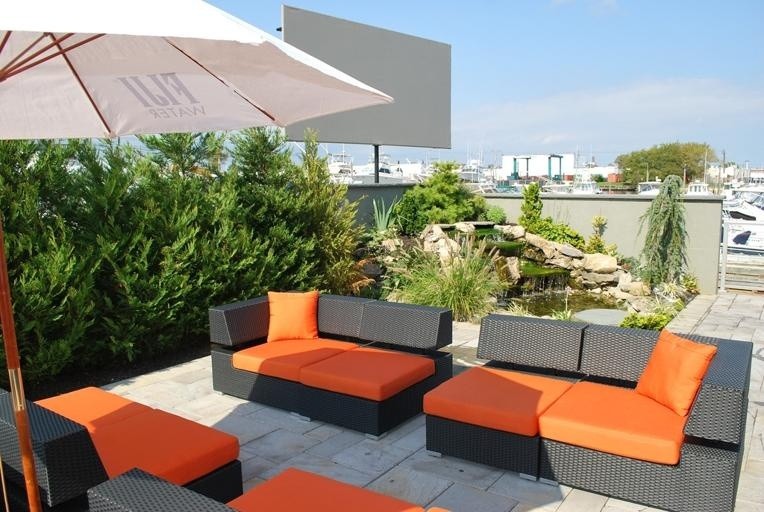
[207,289,453,442]
[0,385,243,512]
[86,466,448,512]
[421,312,754,512]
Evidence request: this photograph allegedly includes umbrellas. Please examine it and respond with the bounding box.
[0,0,395,512]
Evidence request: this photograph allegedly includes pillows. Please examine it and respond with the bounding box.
[633,326,718,417]
[266,290,320,344]
